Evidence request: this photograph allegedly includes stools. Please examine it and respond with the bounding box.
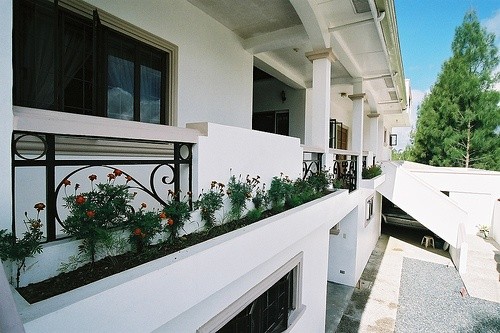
[420,235,436,249]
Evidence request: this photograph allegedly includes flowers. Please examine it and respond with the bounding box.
[362,160,385,178]
[0,169,338,261]
